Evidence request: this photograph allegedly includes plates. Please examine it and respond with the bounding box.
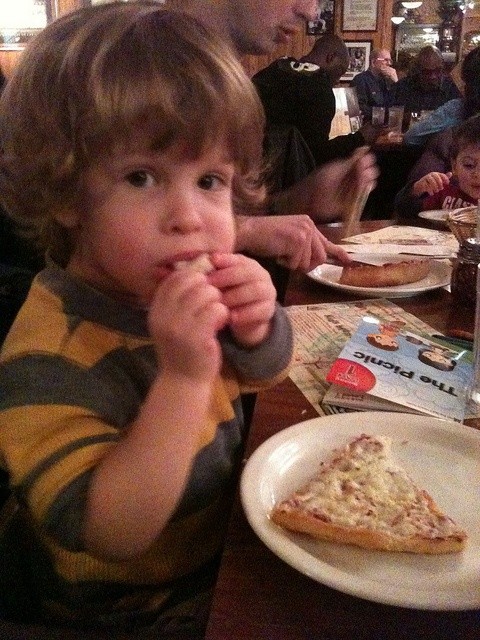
[305,253,454,299]
[418,209,452,226]
[239,410,479,611]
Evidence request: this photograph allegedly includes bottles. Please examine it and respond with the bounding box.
[446,237,480,341]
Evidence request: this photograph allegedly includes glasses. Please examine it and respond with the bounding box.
[418,68,442,75]
[375,56,393,66]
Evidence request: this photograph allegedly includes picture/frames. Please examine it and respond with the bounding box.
[0,0,56,43]
[341,0,379,33]
[337,40,372,84]
[307,1,335,34]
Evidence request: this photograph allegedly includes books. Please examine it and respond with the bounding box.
[321,315,471,424]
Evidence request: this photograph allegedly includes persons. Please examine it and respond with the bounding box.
[405,47,480,145]
[0,1,293,613]
[154,0,382,272]
[348,48,401,118]
[251,33,378,165]
[399,113,480,226]
[402,46,463,130]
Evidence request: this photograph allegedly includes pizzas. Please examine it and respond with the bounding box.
[172,251,213,276]
[339,239,433,286]
[269,432,469,556]
